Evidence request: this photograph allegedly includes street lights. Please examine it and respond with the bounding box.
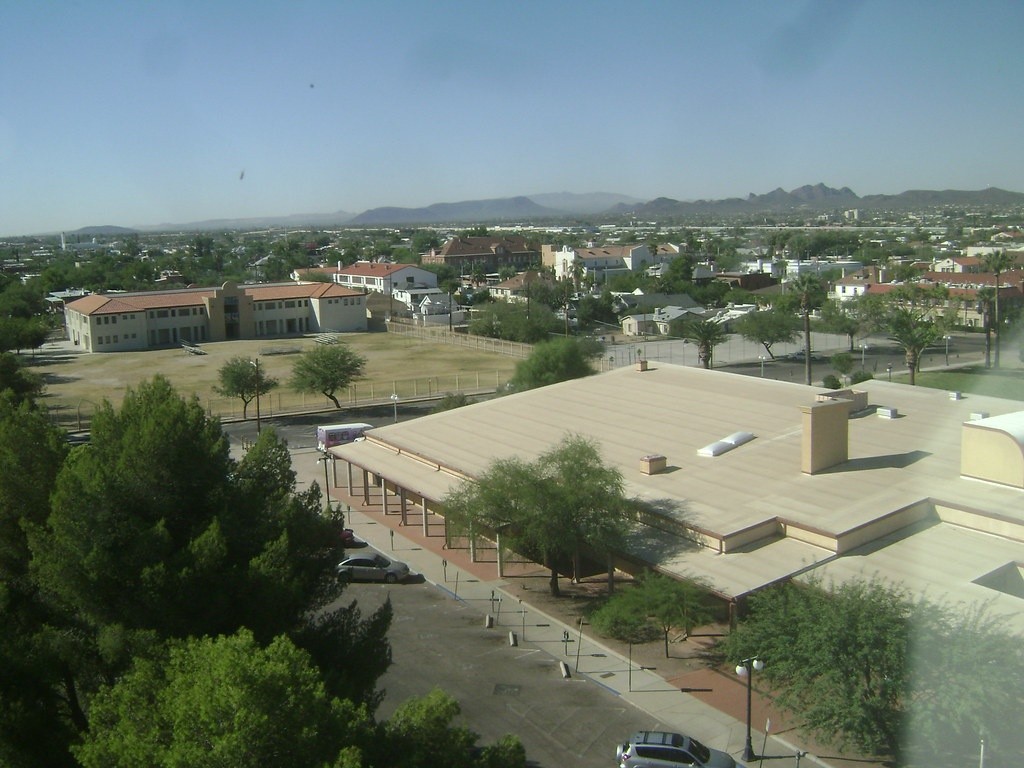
[316,454,334,508]
[942,332,951,366]
[757,354,767,378]
[859,343,868,373]
[391,392,399,424]
[736,653,765,763]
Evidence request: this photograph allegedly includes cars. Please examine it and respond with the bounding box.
[337,553,409,585]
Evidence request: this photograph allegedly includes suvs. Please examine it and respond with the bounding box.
[617,730,736,768]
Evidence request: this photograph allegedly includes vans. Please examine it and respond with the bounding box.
[317,422,375,457]
